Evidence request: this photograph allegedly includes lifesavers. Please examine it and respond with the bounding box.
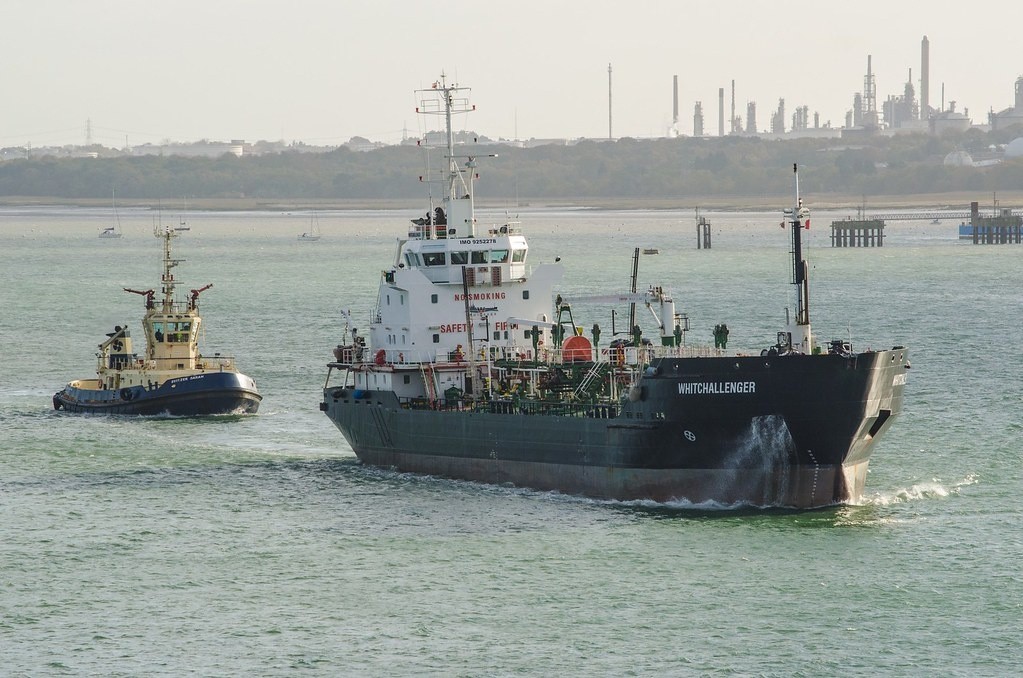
[539,341,543,344]
[120,388,133,401]
[98,378,102,388]
[521,353,526,359]
[374,347,387,366]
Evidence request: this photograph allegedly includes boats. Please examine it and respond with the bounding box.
[97,225,124,239]
[317,63,912,514]
[51,200,263,418]
[173,214,191,231]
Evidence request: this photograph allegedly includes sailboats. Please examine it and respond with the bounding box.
[295,215,321,241]
